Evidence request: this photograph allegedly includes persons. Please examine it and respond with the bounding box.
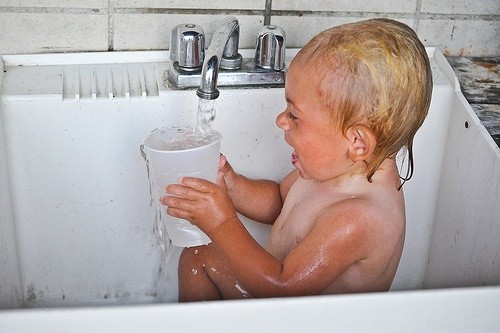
[158,18,432,303]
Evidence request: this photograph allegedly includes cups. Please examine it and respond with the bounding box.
[142,130,222,248]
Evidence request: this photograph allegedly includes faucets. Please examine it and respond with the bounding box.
[196,16,240,101]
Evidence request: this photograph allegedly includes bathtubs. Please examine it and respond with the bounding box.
[0,83,499,311]
